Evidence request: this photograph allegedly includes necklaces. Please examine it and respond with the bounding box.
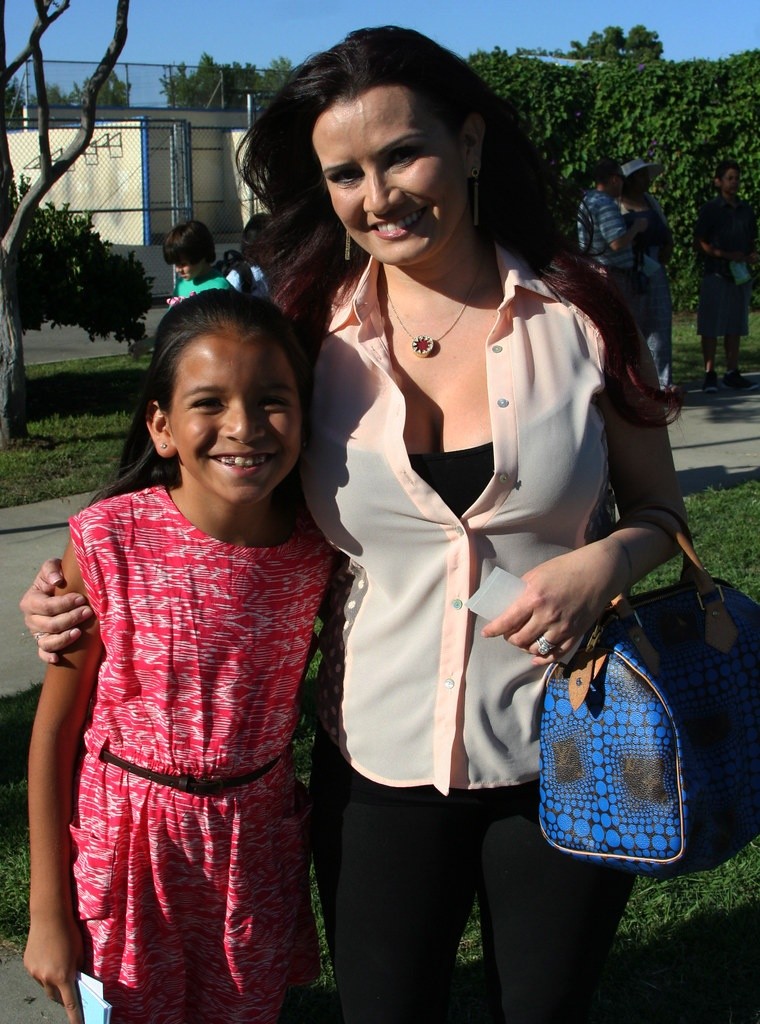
[384,246,485,359]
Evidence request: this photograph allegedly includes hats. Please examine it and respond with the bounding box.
[621,160,665,182]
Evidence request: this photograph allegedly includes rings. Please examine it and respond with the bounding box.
[536,636,555,656]
[33,634,43,641]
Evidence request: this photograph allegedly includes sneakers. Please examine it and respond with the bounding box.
[722,371,759,391]
[704,370,718,392]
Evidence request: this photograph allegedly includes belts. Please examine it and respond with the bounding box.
[98,748,281,795]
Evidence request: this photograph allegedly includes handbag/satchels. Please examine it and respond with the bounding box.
[539,506,760,874]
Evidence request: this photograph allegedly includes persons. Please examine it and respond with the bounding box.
[691,161,760,393]
[578,156,683,393]
[161,213,272,309]
[18,26,691,1024]
[22,289,338,1024]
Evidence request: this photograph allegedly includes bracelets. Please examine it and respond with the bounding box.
[614,537,635,590]
